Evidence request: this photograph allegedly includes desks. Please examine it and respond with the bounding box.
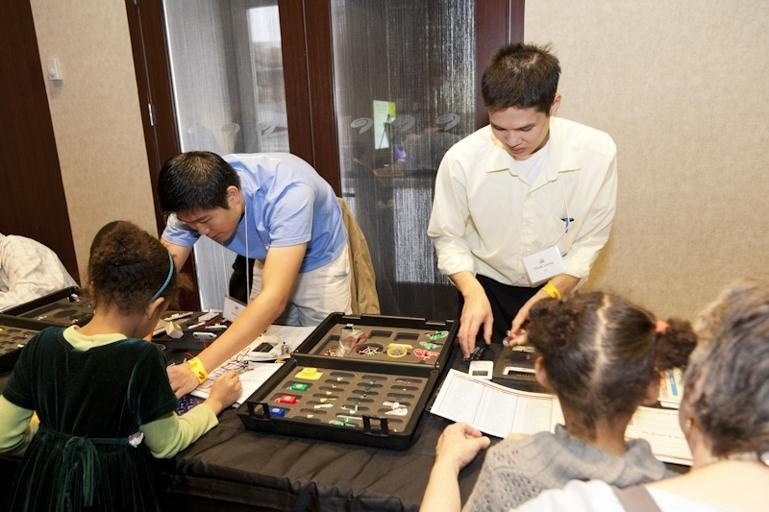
[146,340,692,512]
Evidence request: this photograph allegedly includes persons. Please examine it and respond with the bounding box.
[462,292,698,512]
[0,222,242,512]
[0,233,80,313]
[159,152,352,400]
[419,276,769,512]
[428,46,618,359]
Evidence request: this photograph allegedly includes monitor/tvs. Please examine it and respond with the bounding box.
[371,97,398,151]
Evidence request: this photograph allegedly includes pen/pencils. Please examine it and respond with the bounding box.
[669,370,677,395]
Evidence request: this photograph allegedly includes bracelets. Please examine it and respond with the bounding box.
[542,281,561,300]
[186,357,209,384]
